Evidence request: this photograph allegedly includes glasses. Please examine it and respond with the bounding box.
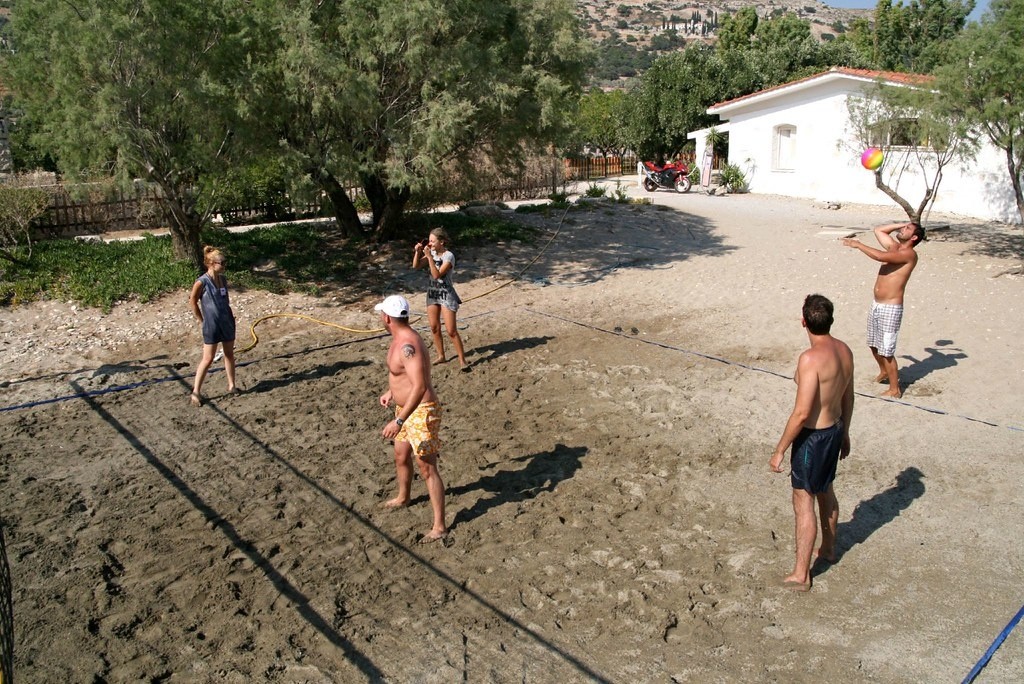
[214,260,226,266]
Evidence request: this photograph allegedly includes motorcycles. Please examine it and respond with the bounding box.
[638,159,693,195]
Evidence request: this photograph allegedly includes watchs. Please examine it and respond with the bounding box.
[395,418,403,425]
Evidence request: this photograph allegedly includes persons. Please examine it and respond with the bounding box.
[374,295,447,543]
[413,228,469,370]
[840,222,924,397]
[191,246,240,407]
[769,294,855,591]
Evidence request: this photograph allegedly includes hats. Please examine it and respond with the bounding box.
[375,294,409,318]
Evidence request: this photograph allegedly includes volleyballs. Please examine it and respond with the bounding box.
[861,147,883,170]
